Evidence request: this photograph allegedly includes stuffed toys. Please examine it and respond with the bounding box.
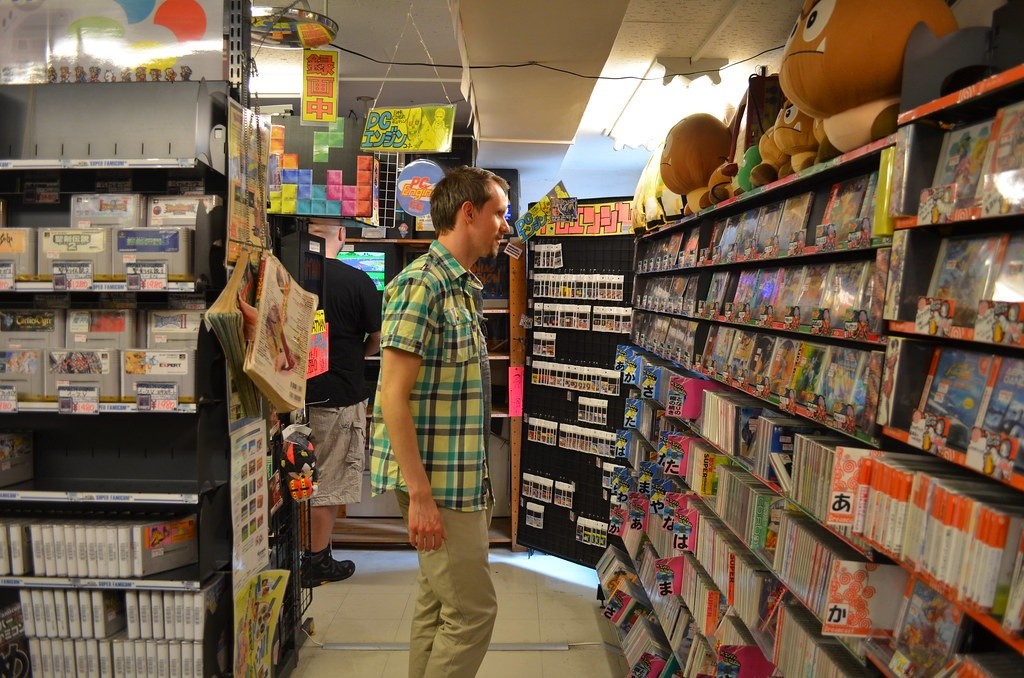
[737,145,762,192]
[773,99,825,179]
[749,125,790,188]
[779,0,959,166]
[660,113,739,216]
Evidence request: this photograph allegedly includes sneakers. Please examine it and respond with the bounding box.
[301,547,355,588]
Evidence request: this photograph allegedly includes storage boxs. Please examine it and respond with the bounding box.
[63,309,136,349]
[113,228,192,280]
[45,347,119,402]
[0,227,35,282]
[147,309,203,351]
[119,348,193,404]
[35,227,111,282]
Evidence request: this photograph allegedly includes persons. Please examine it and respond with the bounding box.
[370,166,511,678]
[298,220,382,587]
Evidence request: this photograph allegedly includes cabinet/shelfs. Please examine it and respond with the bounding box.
[0,0,1024,678]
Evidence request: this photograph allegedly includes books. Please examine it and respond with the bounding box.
[204,249,319,412]
[596,389,1024,678]
